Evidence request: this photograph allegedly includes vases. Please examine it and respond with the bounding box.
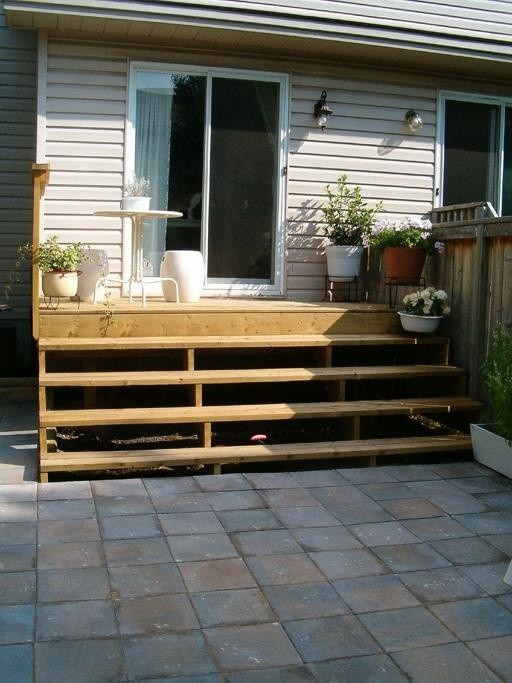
[396,310,445,334]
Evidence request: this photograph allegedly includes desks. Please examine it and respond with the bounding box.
[93,209,185,305]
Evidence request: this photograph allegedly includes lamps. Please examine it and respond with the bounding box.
[405,109,424,134]
[313,89,334,132]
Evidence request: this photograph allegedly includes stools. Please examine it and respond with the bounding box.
[73,247,207,307]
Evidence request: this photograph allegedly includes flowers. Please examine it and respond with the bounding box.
[360,213,460,316]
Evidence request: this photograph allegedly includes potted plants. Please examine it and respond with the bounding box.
[466,317,512,481]
[120,175,152,210]
[19,233,94,299]
[318,173,385,282]
